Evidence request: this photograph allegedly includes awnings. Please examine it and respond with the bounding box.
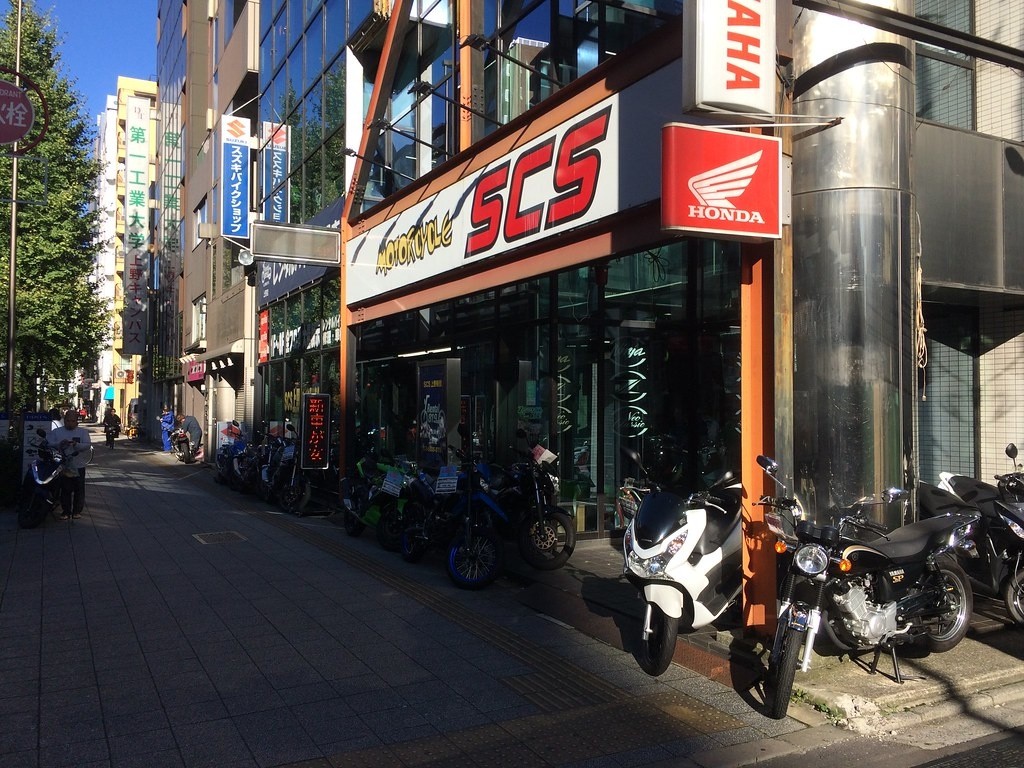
[104,386,114,400]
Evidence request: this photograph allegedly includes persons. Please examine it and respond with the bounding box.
[177,414,202,455]
[76,404,87,422]
[160,408,174,452]
[102,409,121,446]
[48,410,92,519]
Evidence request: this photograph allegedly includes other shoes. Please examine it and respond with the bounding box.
[106,441,109,446]
[73,513,81,519]
[60,511,70,520]
[161,451,171,455]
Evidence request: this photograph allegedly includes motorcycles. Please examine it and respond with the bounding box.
[618,444,744,676]
[919,441,1024,631]
[337,419,578,592]
[215,420,258,493]
[752,454,983,719]
[250,424,312,514]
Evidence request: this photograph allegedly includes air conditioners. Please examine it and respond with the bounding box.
[115,370,127,379]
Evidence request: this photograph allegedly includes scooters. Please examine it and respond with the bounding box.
[15,427,80,530]
[103,422,121,450]
[167,427,192,464]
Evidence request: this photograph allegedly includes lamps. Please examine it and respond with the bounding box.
[458,35,565,88]
[408,81,505,127]
[341,147,415,181]
[237,248,254,267]
[366,118,453,157]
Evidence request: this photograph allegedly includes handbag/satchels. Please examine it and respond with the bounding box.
[118,426,121,432]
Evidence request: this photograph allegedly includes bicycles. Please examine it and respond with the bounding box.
[126,416,144,442]
